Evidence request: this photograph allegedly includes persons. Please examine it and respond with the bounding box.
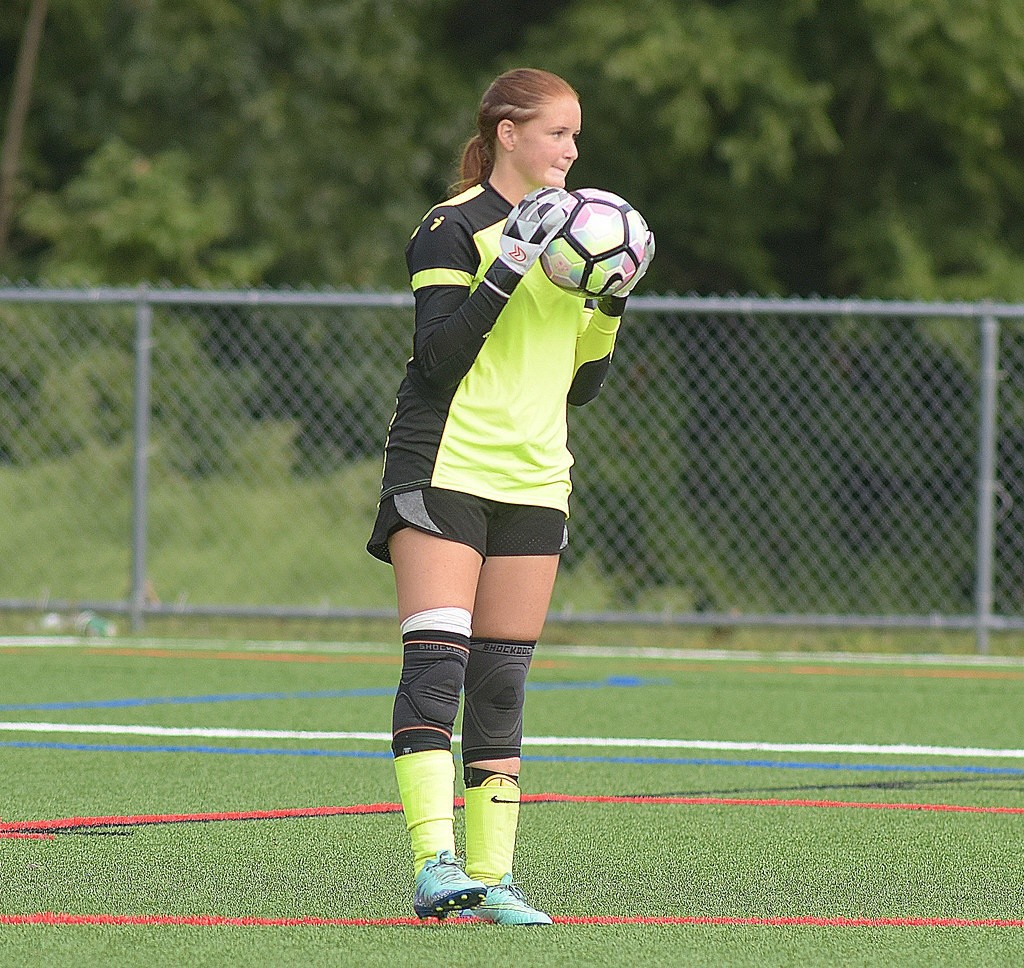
[368,68,656,926]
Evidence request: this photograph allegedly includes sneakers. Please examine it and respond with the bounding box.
[412,851,489,921]
[458,874,554,926]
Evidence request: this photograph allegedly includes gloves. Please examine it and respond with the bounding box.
[609,232,656,300]
[482,185,579,299]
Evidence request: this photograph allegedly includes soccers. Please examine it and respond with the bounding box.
[537,185,651,299]
